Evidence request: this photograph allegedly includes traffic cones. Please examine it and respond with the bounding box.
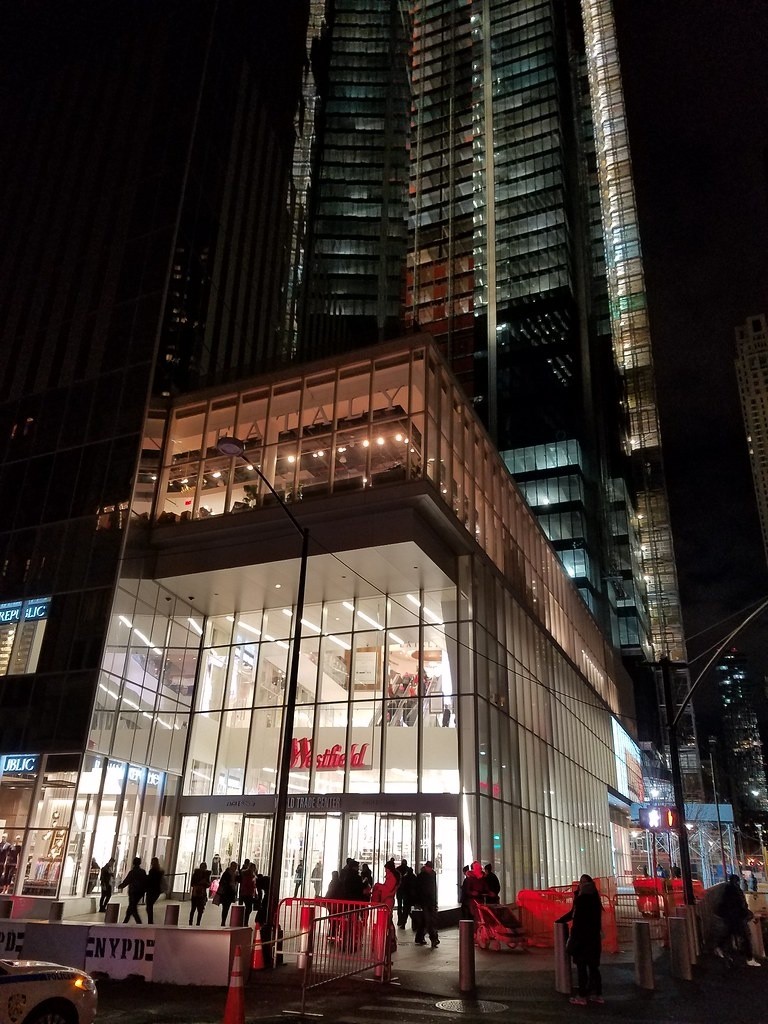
[250,920,265,971]
[222,943,245,1024]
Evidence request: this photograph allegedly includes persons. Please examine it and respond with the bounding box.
[87,855,165,924]
[387,670,431,723]
[293,860,302,897]
[442,704,451,727]
[188,854,269,925]
[671,863,681,880]
[312,863,322,898]
[720,875,761,967]
[740,874,758,892]
[642,865,650,878]
[26,858,32,876]
[324,858,440,955]
[656,864,669,878]
[0,834,21,895]
[436,844,442,874]
[556,875,605,1005]
[457,861,501,946]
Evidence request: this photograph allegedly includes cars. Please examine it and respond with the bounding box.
[0,957,98,1024]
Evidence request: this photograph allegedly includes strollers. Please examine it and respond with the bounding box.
[472,892,532,953]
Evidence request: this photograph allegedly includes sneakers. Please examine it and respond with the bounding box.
[589,995,605,1004]
[569,995,587,1006]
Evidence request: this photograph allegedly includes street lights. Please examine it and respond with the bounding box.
[213,438,313,967]
[707,735,728,884]
[650,788,660,804]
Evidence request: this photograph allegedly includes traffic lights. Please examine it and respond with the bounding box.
[637,805,678,832]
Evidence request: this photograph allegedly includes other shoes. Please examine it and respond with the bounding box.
[431,940,440,948]
[415,937,427,944]
[400,925,405,930]
[747,959,761,967]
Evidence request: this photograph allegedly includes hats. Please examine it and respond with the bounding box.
[730,874,740,884]
[484,863,492,870]
[424,861,433,868]
[463,865,469,871]
[384,861,395,870]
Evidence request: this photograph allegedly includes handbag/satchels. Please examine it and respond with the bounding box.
[212,892,222,906]
